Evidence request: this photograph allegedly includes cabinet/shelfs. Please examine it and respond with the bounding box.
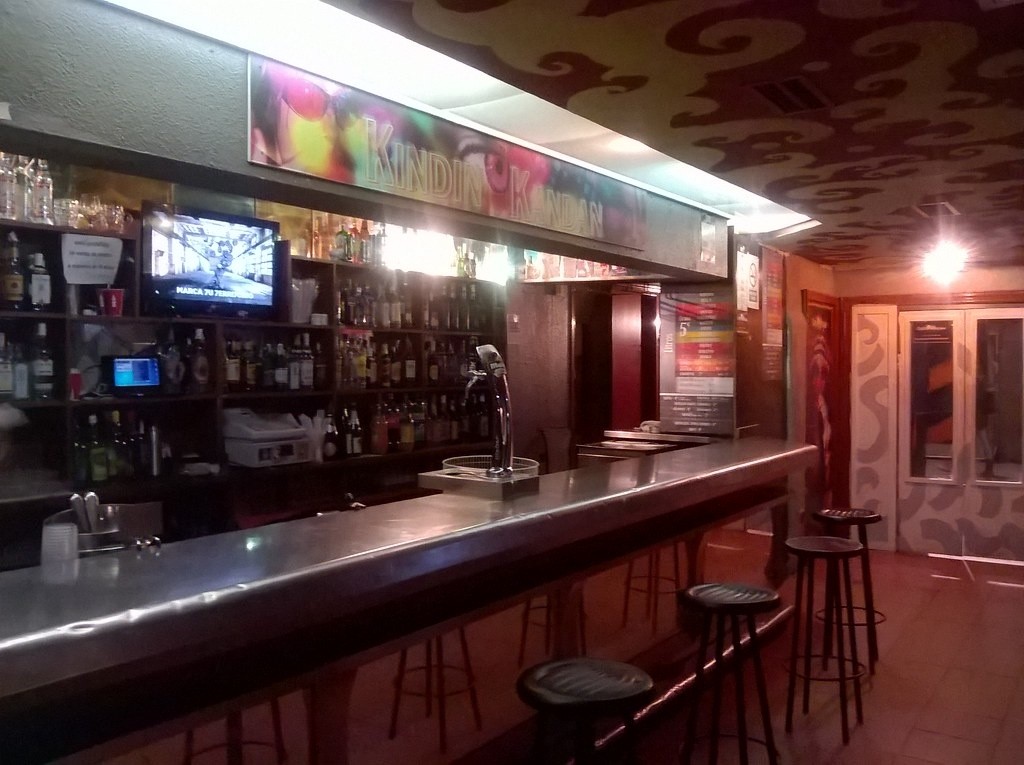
[0,217,505,503]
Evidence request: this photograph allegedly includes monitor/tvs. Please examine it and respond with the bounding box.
[100,354,164,394]
[140,198,281,321]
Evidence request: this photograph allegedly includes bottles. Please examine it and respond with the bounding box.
[0,152,53,225]
[0,322,55,400]
[159,328,210,398]
[1,232,53,312]
[71,408,160,489]
[227,277,491,462]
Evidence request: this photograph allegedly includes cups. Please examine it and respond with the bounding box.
[97,288,125,316]
[54,194,125,232]
[70,368,82,400]
[41,522,80,583]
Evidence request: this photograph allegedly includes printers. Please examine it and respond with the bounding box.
[221,407,314,468]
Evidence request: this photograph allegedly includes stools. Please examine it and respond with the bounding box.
[183,701,289,765]
[516,656,653,765]
[387,627,483,754]
[622,544,682,637]
[785,536,866,745]
[679,582,781,765]
[520,586,587,656]
[815,507,887,675]
